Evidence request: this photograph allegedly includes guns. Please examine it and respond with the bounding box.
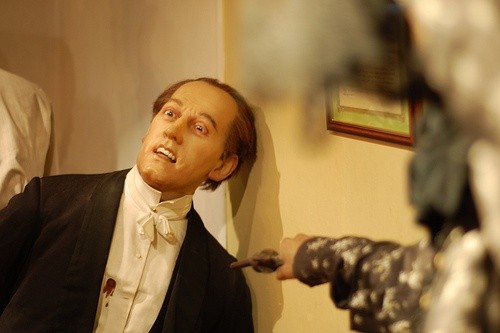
[230,246,283,272]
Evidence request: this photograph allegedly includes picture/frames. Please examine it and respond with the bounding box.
[326,9,423,147]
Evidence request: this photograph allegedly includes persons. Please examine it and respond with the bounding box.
[1,77,261,333]
[271,230,500,333]
